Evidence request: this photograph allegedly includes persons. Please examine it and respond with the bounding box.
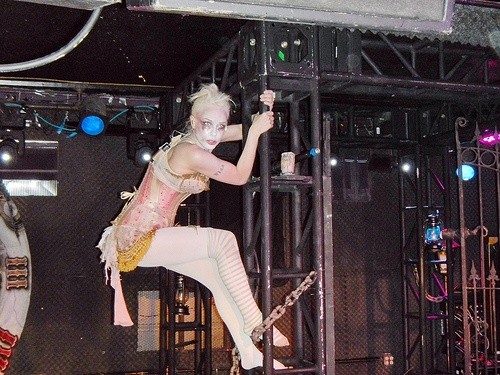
[95,83,290,370]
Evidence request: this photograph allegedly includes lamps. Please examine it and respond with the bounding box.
[77,111,105,137]
[426,215,442,253]
[368,148,399,173]
[126,134,157,166]
[175,275,190,315]
[0,119,25,162]
